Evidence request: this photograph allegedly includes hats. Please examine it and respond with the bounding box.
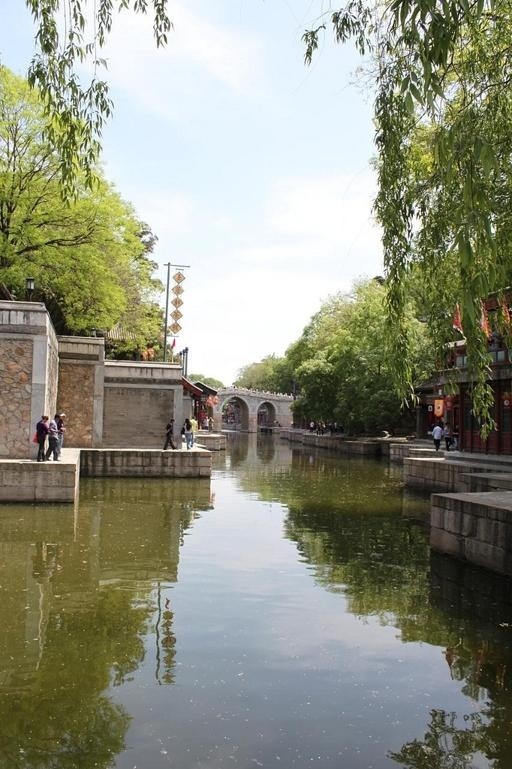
[60,413,66,418]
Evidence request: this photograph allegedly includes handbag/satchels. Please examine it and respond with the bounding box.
[181,427,185,434]
[32,432,39,444]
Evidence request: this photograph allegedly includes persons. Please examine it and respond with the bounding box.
[164,416,214,450]
[432,422,460,451]
[309,420,315,432]
[37,413,67,461]
